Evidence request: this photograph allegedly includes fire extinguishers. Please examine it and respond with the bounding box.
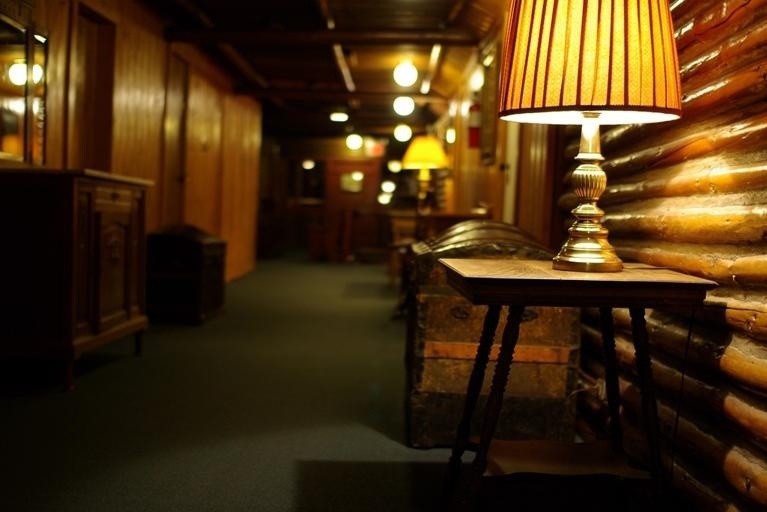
[468,98,482,149]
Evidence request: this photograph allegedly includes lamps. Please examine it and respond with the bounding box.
[497,0,682,273]
[400,136,449,215]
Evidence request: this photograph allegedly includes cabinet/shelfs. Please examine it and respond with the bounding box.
[404,218,582,450]
[0,167,154,394]
[147,224,228,327]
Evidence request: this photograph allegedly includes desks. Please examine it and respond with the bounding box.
[438,257,719,511]
